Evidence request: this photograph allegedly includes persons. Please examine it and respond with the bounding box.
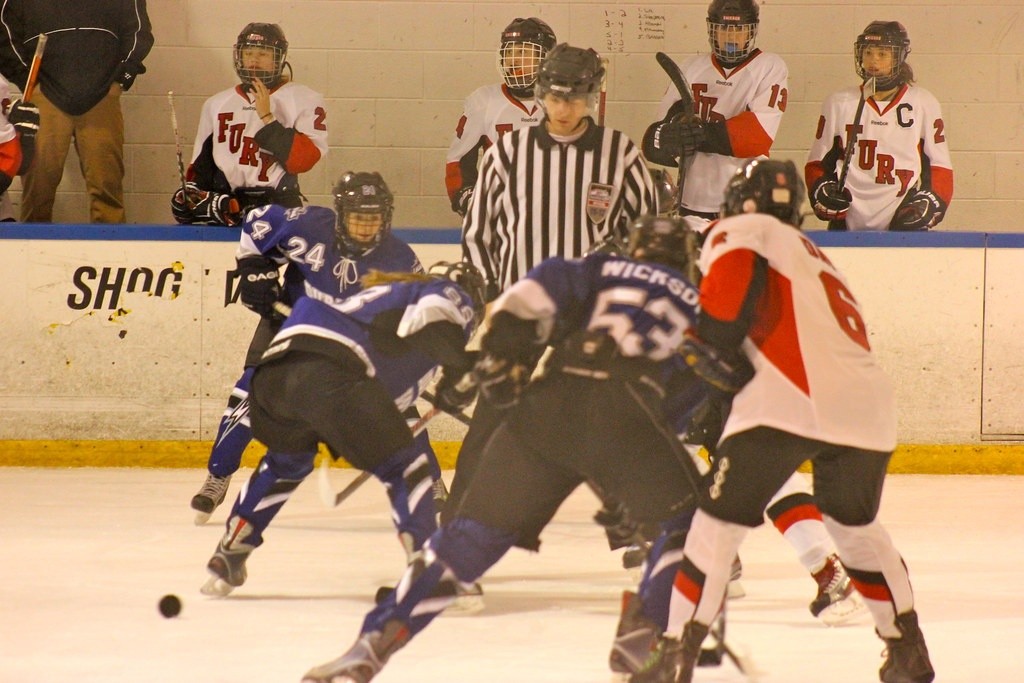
[200,261,484,615]
[189,170,425,523]
[0,77,40,222]
[169,22,328,228]
[442,43,659,530]
[445,18,557,215]
[642,0,790,230]
[0,0,154,224]
[304,159,935,683]
[805,20,954,231]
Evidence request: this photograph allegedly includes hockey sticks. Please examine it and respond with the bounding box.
[659,420,746,582]
[168,89,192,226]
[585,479,757,674]
[597,58,610,129]
[21,31,49,105]
[655,50,697,215]
[272,300,474,426]
[314,320,545,514]
[826,75,878,232]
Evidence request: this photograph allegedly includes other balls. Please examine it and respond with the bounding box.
[159,595,181,617]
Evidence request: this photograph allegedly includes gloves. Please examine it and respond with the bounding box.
[451,184,475,218]
[663,331,746,447]
[8,99,41,148]
[437,364,480,413]
[473,312,532,412]
[645,108,703,167]
[236,254,284,315]
[889,187,946,232]
[808,174,852,221]
[191,190,242,227]
[170,182,201,223]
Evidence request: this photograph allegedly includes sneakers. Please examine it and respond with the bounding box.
[431,479,451,527]
[810,554,868,625]
[594,499,744,683]
[876,611,934,683]
[399,530,486,615]
[201,514,253,598]
[302,620,412,683]
[192,472,232,525]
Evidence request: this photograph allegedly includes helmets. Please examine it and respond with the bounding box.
[854,20,911,91]
[625,215,696,276]
[537,42,606,99]
[498,18,557,94]
[725,156,806,229]
[232,22,289,93]
[427,260,487,325]
[332,171,393,253]
[706,0,760,69]
